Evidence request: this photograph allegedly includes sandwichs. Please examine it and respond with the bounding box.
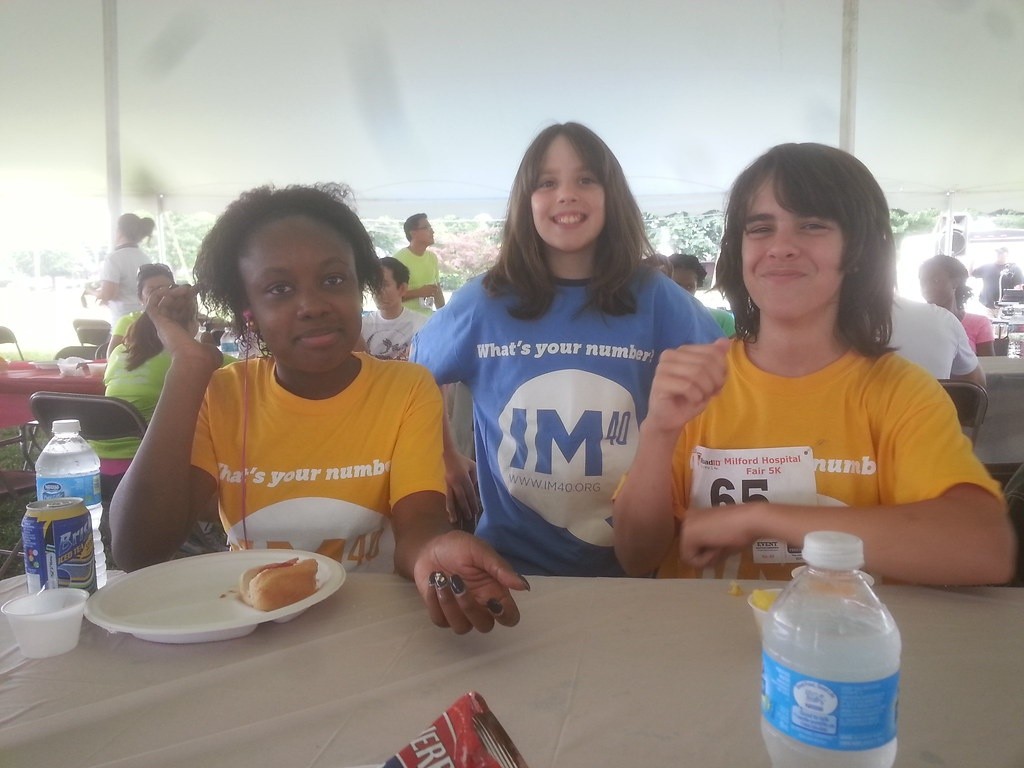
[240,557,320,613]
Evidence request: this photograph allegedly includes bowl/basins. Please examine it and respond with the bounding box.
[0,587,89,659]
[57,363,78,376]
[28,360,94,370]
[86,363,107,376]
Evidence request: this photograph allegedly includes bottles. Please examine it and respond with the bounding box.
[425,297,434,308]
[220,327,259,359]
[196,327,207,343]
[35,419,108,590]
[760,530,902,768]
[1007,309,1024,360]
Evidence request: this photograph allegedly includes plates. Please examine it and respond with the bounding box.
[84,548,346,644]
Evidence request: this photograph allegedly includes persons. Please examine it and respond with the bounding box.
[641,253,737,339]
[394,213,446,318]
[106,263,176,362]
[970,245,1024,322]
[407,123,728,578]
[611,143,1014,585]
[917,254,996,359]
[87,285,259,502]
[109,182,531,636]
[352,257,432,362]
[874,289,987,390]
[99,213,156,336]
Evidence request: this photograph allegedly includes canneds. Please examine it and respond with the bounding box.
[22,497,99,597]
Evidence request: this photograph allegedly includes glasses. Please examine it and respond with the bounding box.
[138,264,172,275]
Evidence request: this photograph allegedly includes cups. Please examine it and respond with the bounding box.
[748,588,783,632]
[791,565,876,588]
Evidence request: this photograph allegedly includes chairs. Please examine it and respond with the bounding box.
[933,377,990,453]
[0,390,147,579]
[53,318,113,359]
[0,325,24,361]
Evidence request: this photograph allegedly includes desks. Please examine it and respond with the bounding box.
[0,570,1024,768]
[0,359,108,470]
[952,356,1024,465]
